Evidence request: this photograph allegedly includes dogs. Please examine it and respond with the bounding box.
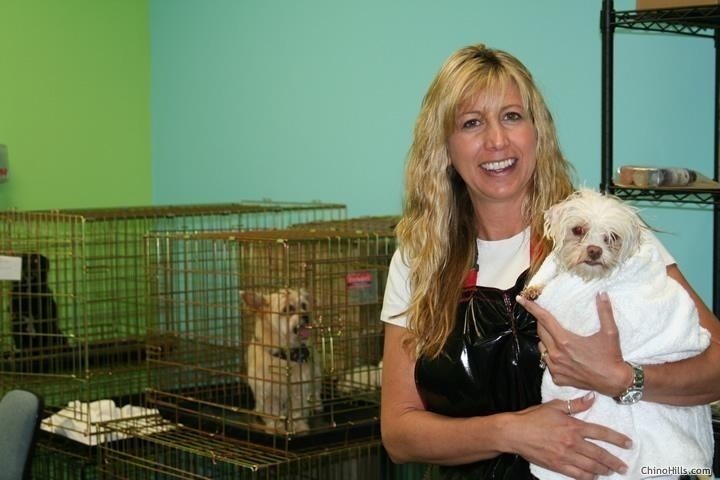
[7,251,70,348]
[237,284,325,436]
[516,185,647,301]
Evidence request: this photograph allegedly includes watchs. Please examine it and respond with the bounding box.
[612,363,644,406]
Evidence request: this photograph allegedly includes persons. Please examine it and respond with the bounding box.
[379,43,720,480]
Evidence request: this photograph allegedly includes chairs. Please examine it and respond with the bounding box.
[1,387,40,479]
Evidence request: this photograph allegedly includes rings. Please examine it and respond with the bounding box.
[568,399,573,415]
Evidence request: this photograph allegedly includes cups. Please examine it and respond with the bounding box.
[616,165,698,187]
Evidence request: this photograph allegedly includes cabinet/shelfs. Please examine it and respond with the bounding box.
[596,0,716,311]
